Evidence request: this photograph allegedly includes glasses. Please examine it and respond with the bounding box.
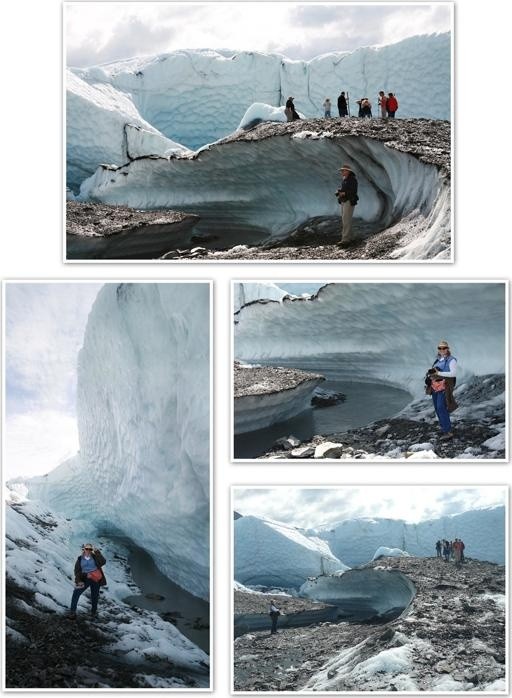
[438,346,448,350]
[84,548,94,551]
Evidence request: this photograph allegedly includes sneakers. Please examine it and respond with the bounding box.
[338,240,346,245]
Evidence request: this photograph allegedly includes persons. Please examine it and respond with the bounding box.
[426,339,458,439]
[269,597,281,633]
[435,537,465,564]
[334,164,359,245]
[283,91,398,121]
[70,541,107,616]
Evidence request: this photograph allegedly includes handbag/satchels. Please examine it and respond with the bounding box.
[87,570,103,584]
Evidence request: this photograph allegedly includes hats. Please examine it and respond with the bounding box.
[438,341,449,348]
[338,163,356,176]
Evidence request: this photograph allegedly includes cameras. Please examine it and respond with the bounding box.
[428,367,441,374]
